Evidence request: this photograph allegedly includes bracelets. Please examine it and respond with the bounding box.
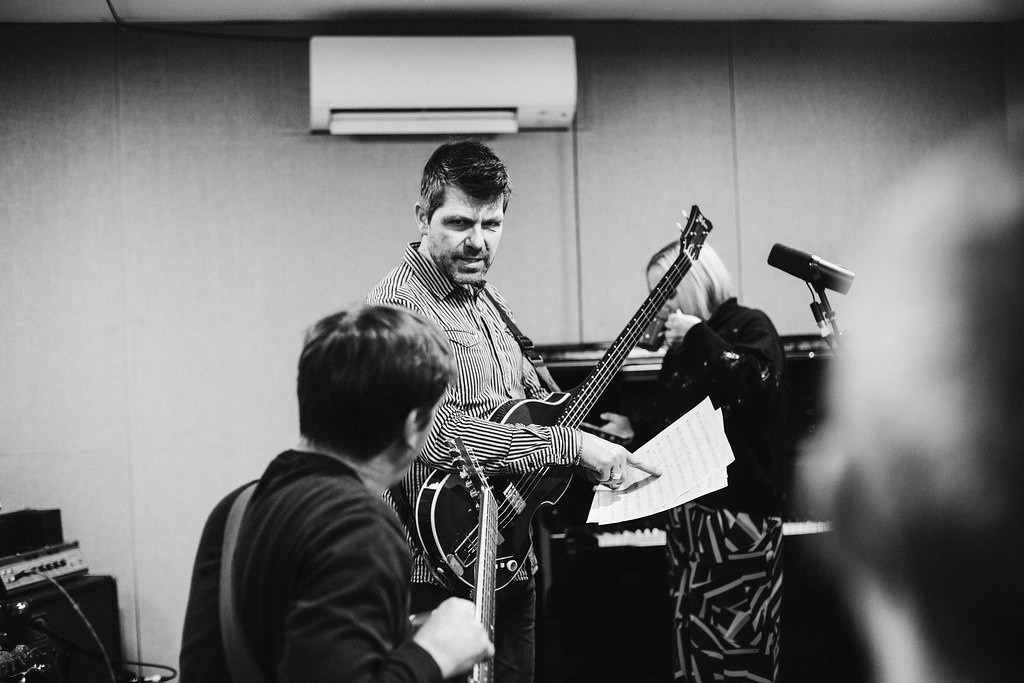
[575,428,585,465]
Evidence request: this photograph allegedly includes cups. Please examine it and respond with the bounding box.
[635,313,668,352]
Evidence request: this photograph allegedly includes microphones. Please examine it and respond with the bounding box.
[125,675,169,683]
[767,243,855,294]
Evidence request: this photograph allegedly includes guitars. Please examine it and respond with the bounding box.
[414,204,714,600]
[453,436,537,683]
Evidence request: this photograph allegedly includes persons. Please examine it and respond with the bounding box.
[363,140,662,683]
[178,305,495,683]
[793,113,1024,683]
[598,240,786,683]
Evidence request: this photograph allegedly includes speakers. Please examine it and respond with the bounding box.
[0,575,124,683]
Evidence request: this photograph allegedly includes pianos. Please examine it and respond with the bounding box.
[526,329,871,683]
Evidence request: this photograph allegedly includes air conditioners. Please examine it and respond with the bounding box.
[307,36,577,137]
